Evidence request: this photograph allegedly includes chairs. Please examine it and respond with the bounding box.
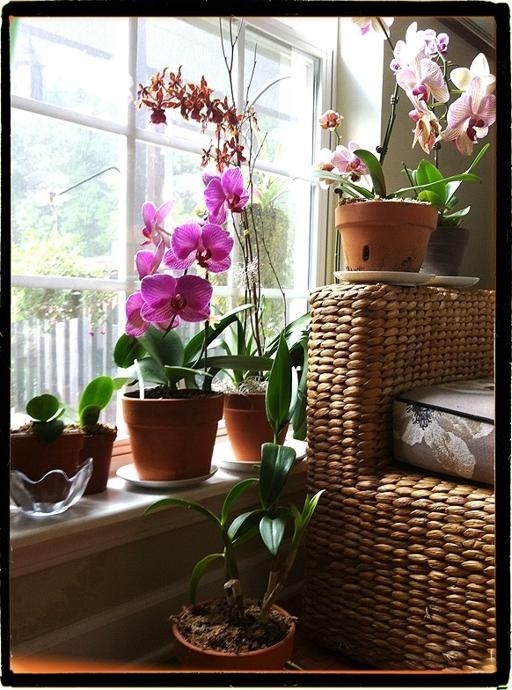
[303,285,499,673]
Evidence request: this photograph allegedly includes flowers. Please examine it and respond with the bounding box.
[314,17,497,224]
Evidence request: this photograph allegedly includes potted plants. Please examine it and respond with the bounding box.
[9,375,131,504]
[134,333,331,671]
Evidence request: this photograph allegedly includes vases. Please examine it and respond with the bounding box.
[118,386,289,481]
[332,204,471,275]
